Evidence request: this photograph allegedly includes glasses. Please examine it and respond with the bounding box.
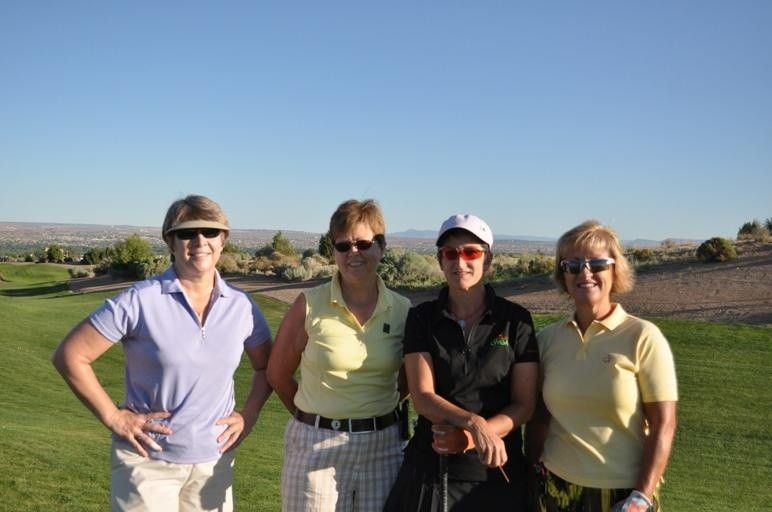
[559,256,615,275]
[176,229,220,240]
[440,246,486,261]
[334,240,375,253]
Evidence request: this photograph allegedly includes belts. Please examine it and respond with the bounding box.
[296,405,401,436]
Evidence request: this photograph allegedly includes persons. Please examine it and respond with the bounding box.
[51,196,275,512]
[524,219,679,512]
[384,214,540,512]
[267,200,414,512]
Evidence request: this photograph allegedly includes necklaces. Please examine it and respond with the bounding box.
[446,298,484,329]
[197,312,202,317]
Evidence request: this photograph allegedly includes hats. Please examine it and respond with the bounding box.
[436,214,493,249]
[164,219,231,234]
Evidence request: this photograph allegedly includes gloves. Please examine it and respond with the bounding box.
[615,490,652,512]
[431,423,476,456]
